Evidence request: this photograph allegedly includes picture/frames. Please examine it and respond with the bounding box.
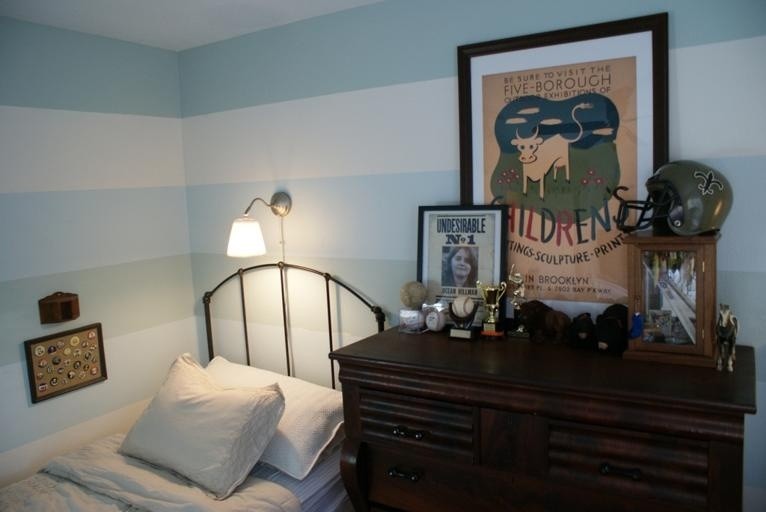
[617,233,719,370]
[415,204,509,337]
[454,12,672,337]
[23,320,108,404]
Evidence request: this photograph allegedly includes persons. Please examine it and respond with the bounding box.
[439,245,479,288]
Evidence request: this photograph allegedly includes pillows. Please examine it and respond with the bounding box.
[115,352,345,503]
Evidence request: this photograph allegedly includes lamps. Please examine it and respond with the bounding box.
[226,190,291,260]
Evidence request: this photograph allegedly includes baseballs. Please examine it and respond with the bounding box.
[451,295,474,318]
[400,281,425,308]
[402,311,424,331]
[426,311,446,332]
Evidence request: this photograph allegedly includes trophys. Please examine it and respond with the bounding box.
[508,262,527,330]
[475,281,507,332]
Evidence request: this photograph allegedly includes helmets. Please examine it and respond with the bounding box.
[616,159,732,237]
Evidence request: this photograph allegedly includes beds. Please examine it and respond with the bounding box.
[0,263,388,511]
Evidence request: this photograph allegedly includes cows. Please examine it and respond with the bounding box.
[511,103,594,201]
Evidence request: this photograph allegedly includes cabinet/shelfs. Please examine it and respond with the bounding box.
[327,325,760,511]
